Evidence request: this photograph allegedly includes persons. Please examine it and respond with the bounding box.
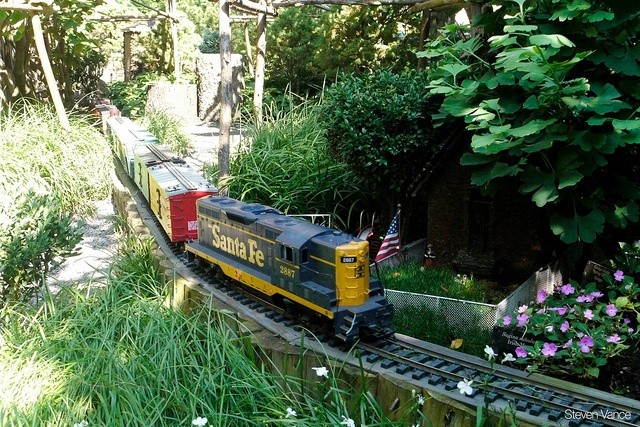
[424,244,436,266]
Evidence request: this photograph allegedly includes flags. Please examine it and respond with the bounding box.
[374,213,400,261]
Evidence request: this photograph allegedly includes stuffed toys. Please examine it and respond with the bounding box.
[91,97,396,346]
